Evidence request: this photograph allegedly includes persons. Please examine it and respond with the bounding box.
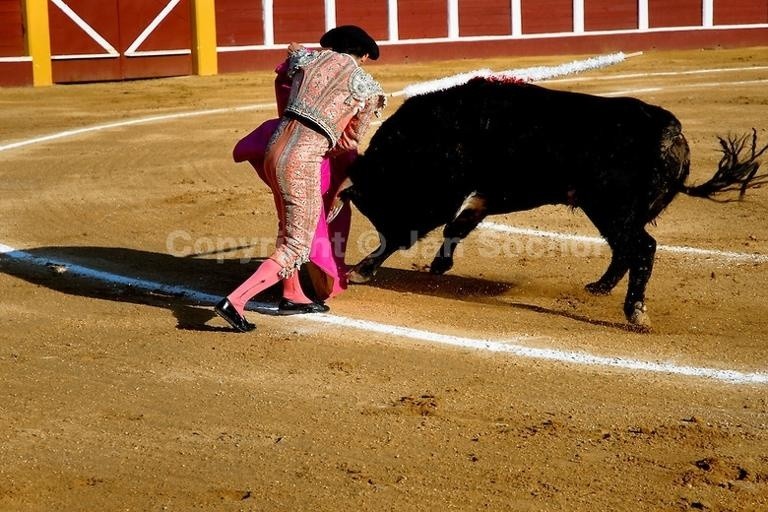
[213,26,386,332]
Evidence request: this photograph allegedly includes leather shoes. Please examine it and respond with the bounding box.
[213,296,256,332]
[275,295,330,316]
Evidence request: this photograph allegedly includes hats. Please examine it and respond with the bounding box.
[319,23,380,60]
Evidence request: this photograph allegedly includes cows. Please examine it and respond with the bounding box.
[336,74,768,329]
